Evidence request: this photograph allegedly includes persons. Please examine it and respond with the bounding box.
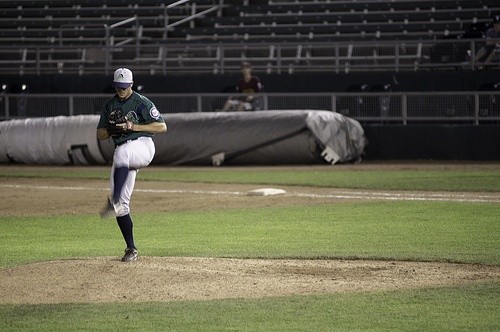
[482,15,500,69]
[220,60,264,110]
[96,68,168,262]
[431,27,469,75]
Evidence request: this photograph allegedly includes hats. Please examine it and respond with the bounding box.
[112,68,134,89]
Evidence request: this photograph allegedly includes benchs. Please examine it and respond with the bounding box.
[0,0,500,131]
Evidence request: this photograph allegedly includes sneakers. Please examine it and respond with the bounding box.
[99,195,120,217]
[122,247,138,262]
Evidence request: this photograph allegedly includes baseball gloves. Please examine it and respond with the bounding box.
[106,108,131,138]
